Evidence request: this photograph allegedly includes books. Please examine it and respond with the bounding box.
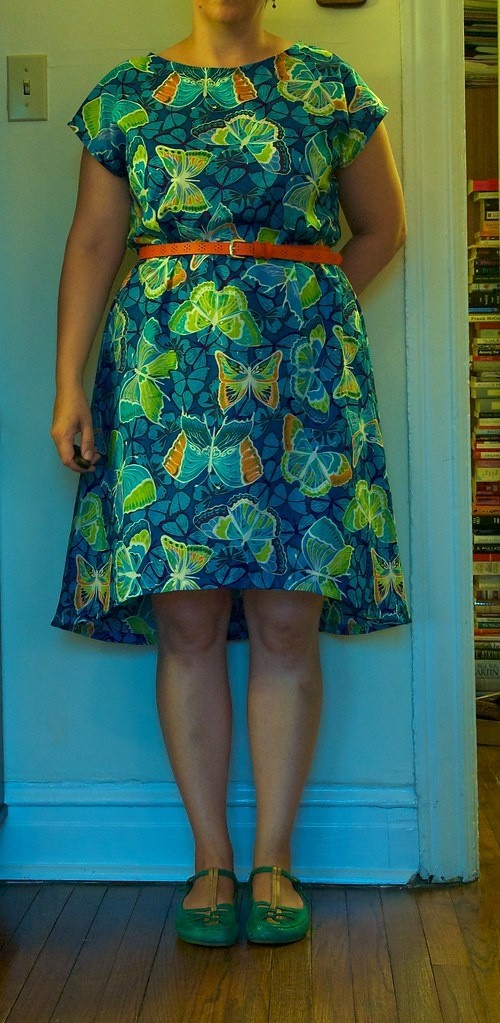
[461,5,499,86]
[466,177,500,746]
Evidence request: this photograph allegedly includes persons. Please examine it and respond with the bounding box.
[50,0,413,947]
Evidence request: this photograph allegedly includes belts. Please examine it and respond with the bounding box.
[139,238,343,265]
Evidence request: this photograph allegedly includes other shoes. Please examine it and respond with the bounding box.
[177,867,239,946]
[246,867,310,944]
